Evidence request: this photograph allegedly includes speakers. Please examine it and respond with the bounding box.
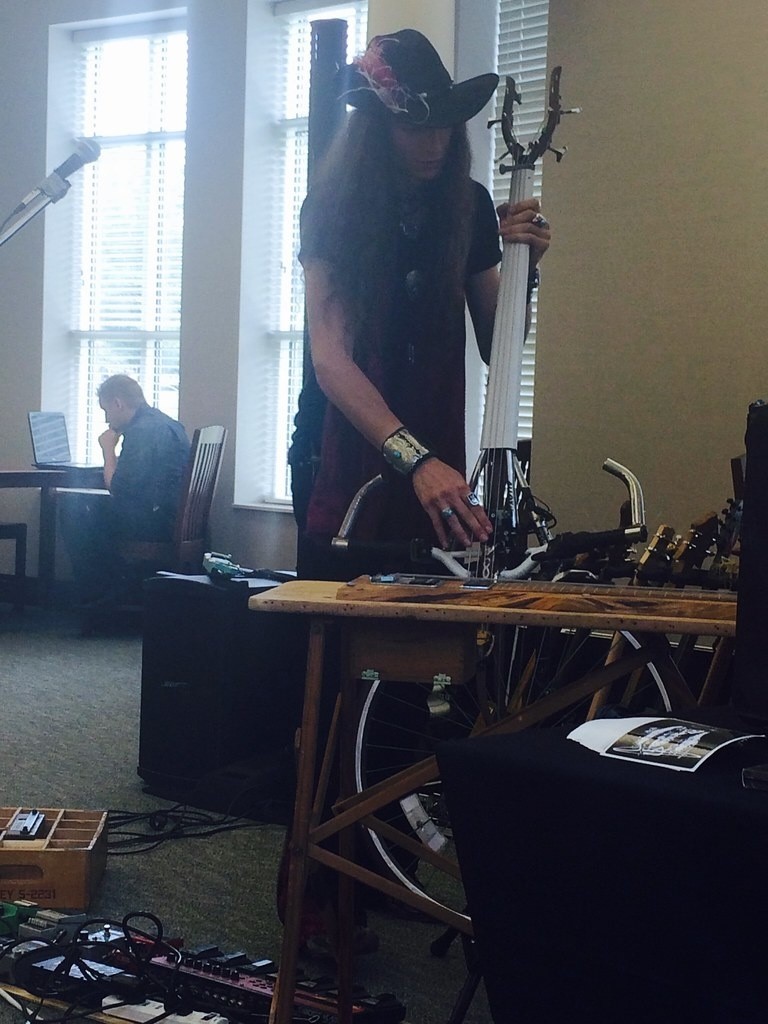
[139,572,308,822]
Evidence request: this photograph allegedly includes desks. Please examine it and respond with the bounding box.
[249,581,737,1024]
[0,470,107,641]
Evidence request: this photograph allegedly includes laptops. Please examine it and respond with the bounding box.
[27,412,104,470]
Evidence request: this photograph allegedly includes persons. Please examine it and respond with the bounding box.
[276,28,550,955]
[63,375,191,610]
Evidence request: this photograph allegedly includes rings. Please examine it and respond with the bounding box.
[466,491,481,508]
[533,212,545,228]
[441,509,453,520]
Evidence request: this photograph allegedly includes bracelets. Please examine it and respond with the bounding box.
[527,267,540,304]
[381,427,430,479]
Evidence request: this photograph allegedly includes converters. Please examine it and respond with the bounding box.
[31,955,127,1005]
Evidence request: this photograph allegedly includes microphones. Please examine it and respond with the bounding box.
[13,138,102,216]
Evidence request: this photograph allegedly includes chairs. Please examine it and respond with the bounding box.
[80,425,229,635]
[0,521,28,616]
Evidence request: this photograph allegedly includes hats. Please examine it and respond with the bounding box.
[331,30,499,128]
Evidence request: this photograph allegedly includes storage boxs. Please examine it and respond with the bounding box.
[0,807,108,909]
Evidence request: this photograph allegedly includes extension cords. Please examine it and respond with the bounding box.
[102,993,229,1024]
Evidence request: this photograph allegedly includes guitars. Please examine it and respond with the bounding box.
[355,66,696,918]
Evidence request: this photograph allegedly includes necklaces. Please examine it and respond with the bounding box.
[394,179,428,304]
